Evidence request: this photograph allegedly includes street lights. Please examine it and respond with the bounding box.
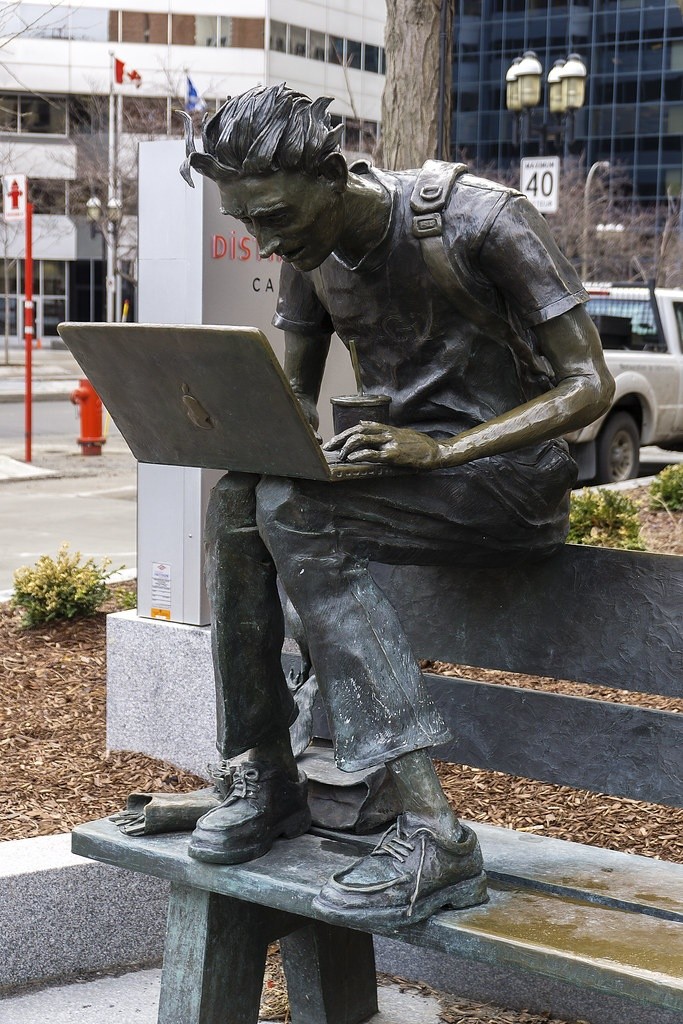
[84,195,126,323]
[506,48,588,159]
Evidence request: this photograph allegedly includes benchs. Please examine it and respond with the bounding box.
[72,546,683,1023]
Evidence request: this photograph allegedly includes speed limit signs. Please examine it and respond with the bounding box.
[520,157,560,216]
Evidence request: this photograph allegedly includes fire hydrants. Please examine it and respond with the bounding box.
[69,373,107,457]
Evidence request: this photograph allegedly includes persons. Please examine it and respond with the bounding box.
[177,82,617,931]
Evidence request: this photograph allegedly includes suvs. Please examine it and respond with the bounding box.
[561,283,683,488]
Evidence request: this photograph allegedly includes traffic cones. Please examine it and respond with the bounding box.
[37,336,42,352]
